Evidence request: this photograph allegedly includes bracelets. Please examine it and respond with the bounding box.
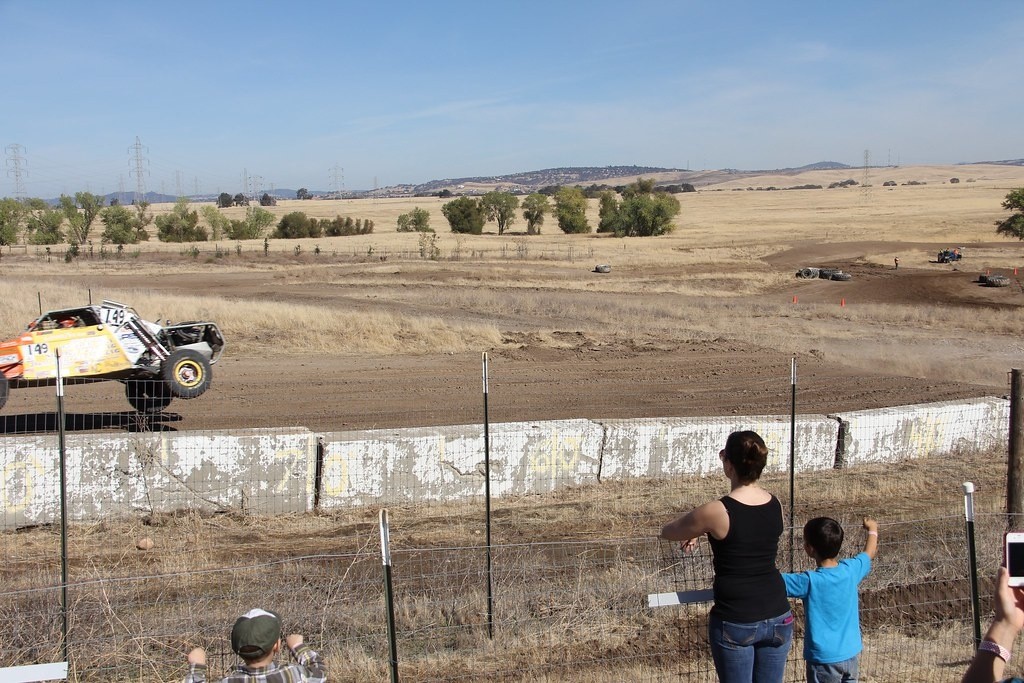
[869,531,877,536]
[980,641,1011,663]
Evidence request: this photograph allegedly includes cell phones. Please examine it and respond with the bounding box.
[1003,530,1024,588]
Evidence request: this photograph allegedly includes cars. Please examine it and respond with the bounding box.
[819,268,851,281]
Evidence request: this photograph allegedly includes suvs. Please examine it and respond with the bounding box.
[938,251,962,263]
[0,298,227,413]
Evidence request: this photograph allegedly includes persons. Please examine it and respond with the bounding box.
[939,248,943,258]
[186,608,326,683]
[894,257,899,269]
[781,518,882,683]
[954,248,958,260]
[945,248,950,263]
[661,430,793,683]
[961,566,1024,683]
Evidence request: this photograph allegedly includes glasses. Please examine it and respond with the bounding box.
[719,450,726,458]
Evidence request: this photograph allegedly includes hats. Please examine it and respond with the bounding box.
[231,606,282,658]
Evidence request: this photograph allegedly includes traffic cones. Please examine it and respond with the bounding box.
[1014,266,1018,275]
[839,297,845,307]
[792,294,797,303]
[987,269,990,277]
